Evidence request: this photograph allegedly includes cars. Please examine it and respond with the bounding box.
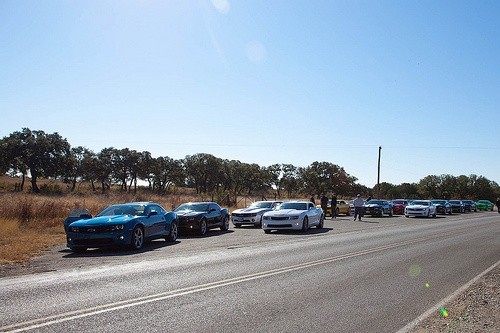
[262,201,325,234]
[391,199,410,215]
[316,200,351,217]
[64,202,177,251]
[431,200,453,215]
[462,199,477,212]
[231,200,285,229]
[364,200,394,218]
[475,200,493,212]
[405,200,436,218]
[349,199,369,215]
[448,200,465,214]
[171,202,233,237]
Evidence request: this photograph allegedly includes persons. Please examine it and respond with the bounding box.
[366,196,371,201]
[495,197,500,213]
[310,194,315,204]
[353,194,363,221]
[321,194,328,219]
[331,193,337,219]
